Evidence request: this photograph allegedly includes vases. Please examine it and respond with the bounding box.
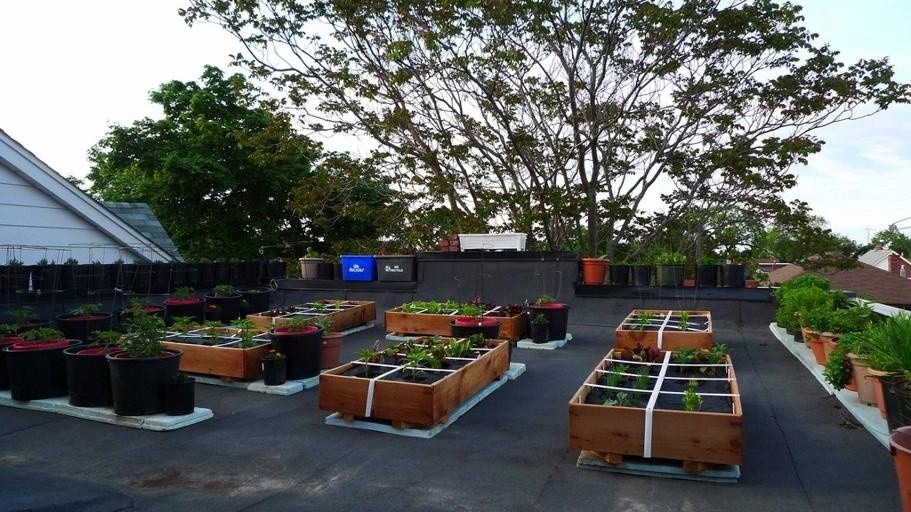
[458,233,528,252]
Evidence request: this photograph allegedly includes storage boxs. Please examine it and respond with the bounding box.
[374,254,415,282]
[339,254,376,281]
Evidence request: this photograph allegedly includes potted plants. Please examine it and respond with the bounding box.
[773,275,911,512]
[719,249,745,288]
[630,258,652,286]
[568,310,743,471]
[63,329,130,407]
[582,258,609,285]
[5,327,83,402]
[683,269,695,287]
[609,262,630,286]
[319,294,568,430]
[300,246,341,282]
[0,305,50,391]
[745,263,769,288]
[655,251,687,286]
[165,370,195,416]
[695,257,718,290]
[58,287,377,387]
[100,295,183,416]
